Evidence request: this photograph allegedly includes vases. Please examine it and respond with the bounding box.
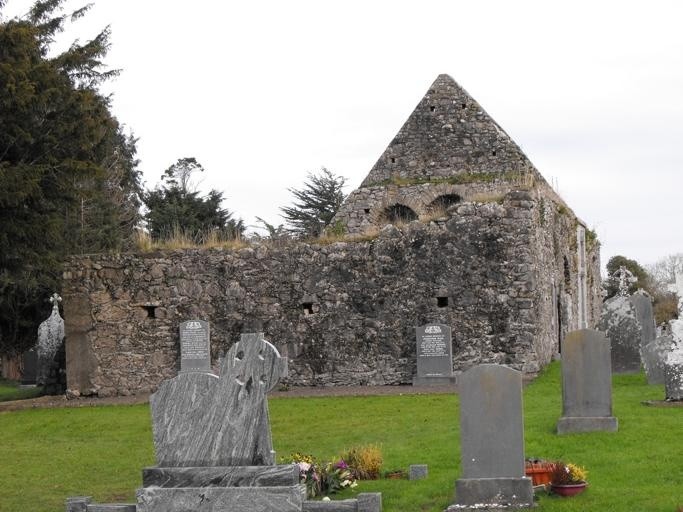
[525,468,550,485]
[550,484,586,498]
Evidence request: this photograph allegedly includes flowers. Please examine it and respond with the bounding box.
[546,461,586,484]
